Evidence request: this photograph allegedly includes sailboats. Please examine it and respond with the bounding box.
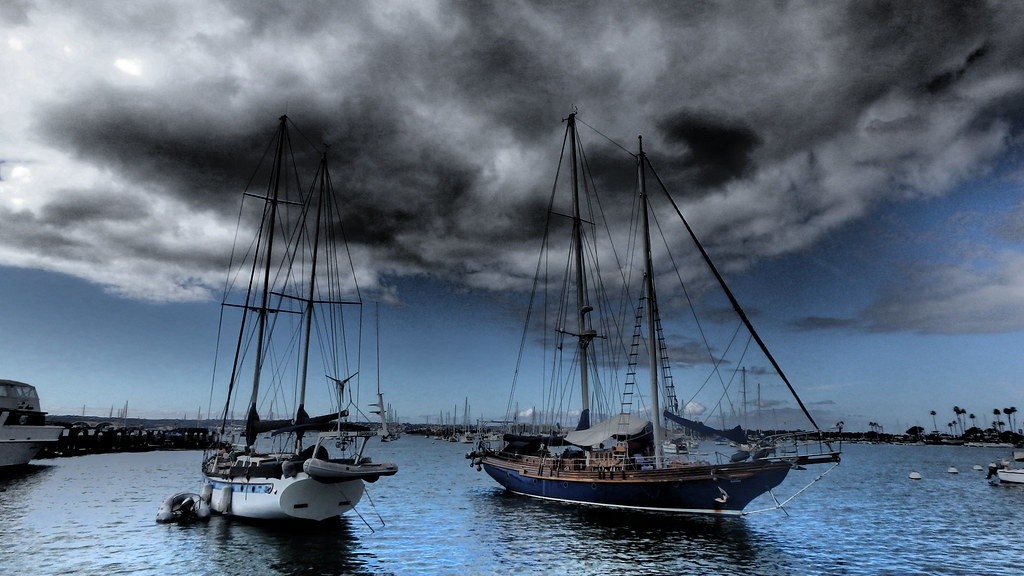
[466,103,844,518]
[197,388,573,444]
[714,366,834,452]
[661,390,700,454]
[198,100,400,525]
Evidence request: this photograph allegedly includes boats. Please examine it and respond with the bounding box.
[963,441,1015,449]
[996,467,1024,484]
[0,379,66,472]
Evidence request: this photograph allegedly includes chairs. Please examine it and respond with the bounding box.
[633,454,654,469]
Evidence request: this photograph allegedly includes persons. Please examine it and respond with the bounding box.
[213,444,226,460]
